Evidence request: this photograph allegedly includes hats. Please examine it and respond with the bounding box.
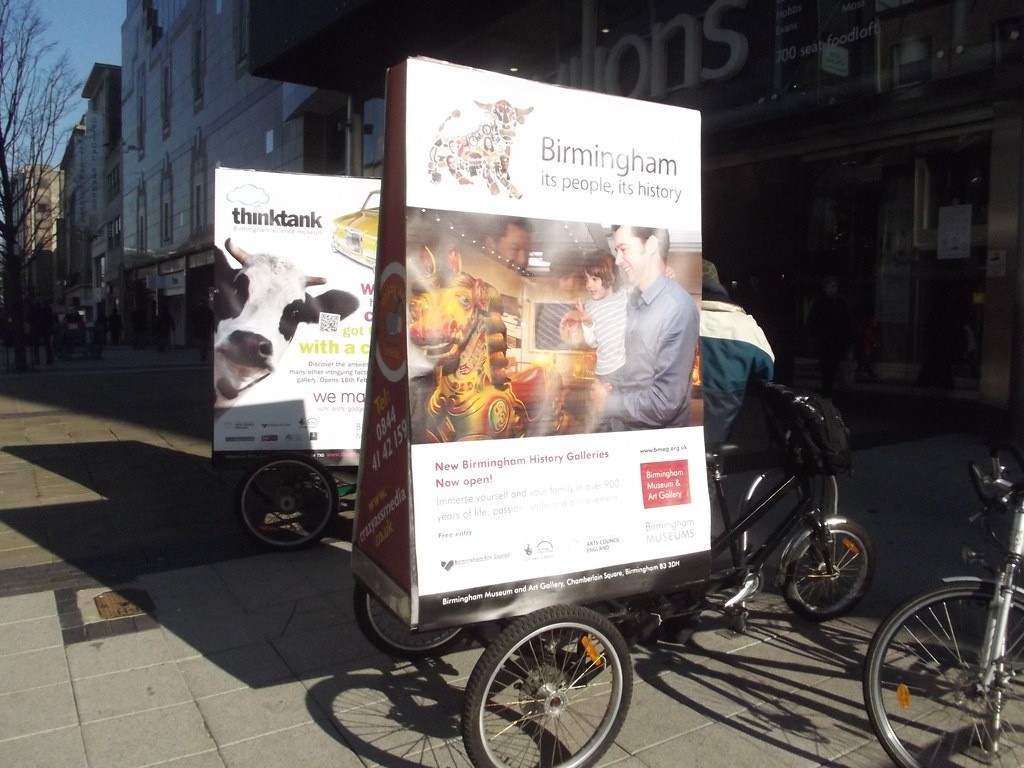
[197,295,208,304]
[702,257,719,283]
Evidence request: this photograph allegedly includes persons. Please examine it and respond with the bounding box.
[27,303,51,366]
[97,311,109,346]
[132,305,146,350]
[535,224,700,432]
[109,308,123,346]
[153,304,175,353]
[190,297,214,361]
[406,212,534,444]
[699,256,776,504]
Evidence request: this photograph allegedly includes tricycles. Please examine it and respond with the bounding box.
[235,447,359,555]
[352,380,875,768]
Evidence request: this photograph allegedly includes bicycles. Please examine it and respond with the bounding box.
[862,439,1024,768]
